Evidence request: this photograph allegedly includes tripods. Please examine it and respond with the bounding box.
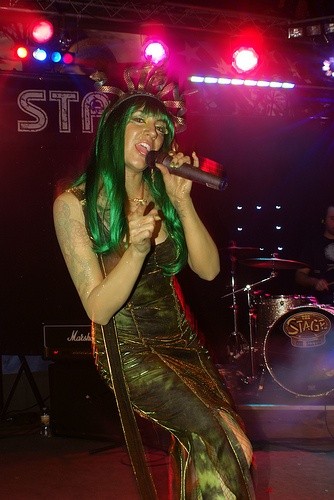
[219,256,277,399]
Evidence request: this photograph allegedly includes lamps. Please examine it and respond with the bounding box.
[0,8,260,75]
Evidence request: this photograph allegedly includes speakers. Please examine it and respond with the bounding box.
[43,324,127,443]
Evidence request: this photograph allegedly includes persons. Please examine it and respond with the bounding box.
[295,203,334,307]
[53,93,257,500]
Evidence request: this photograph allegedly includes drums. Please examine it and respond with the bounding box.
[262,304,334,397]
[251,295,315,353]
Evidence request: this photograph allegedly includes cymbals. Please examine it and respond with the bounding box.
[244,258,307,271]
[222,246,260,254]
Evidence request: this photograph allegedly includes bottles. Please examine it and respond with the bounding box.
[40,407,51,438]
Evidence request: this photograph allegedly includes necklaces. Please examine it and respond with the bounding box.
[128,183,149,206]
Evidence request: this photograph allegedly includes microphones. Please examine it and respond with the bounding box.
[145,149,228,191]
